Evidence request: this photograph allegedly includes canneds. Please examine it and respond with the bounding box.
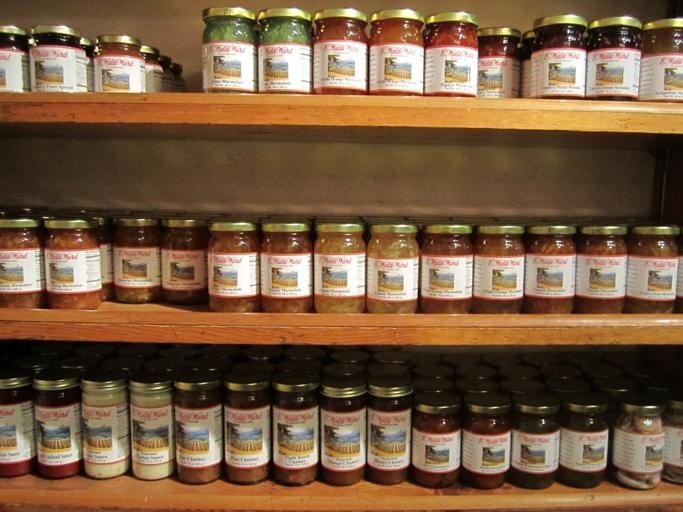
[0,349,683,491]
[1,206,683,315]
[200,5,683,100]
[0,23,185,94]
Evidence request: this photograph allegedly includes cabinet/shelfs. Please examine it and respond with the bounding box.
[0,90,683,512]
